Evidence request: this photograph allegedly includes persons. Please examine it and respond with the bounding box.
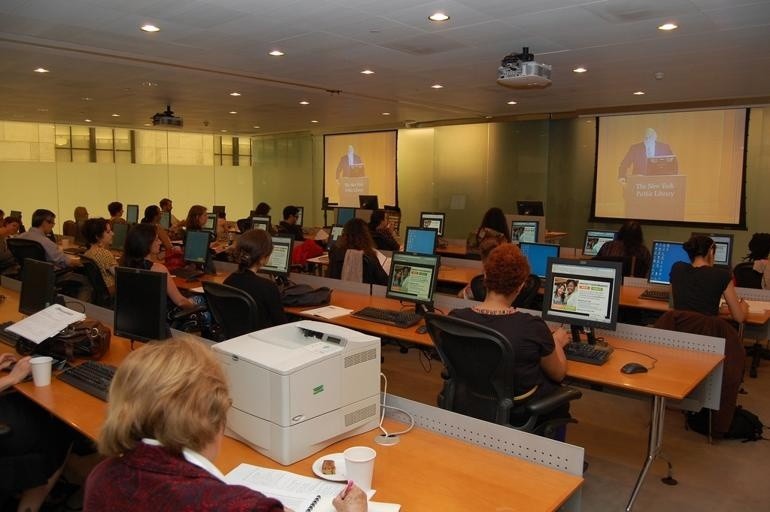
[447,243,589,478]
[733,232,770,290]
[276,205,303,242]
[566,279,578,307]
[222,228,289,327]
[402,266,410,288]
[183,205,218,277]
[0,216,21,277]
[82,340,368,512]
[587,238,599,250]
[618,127,680,182]
[74,207,89,227]
[323,218,392,287]
[16,209,94,303]
[519,227,524,241]
[553,283,565,305]
[475,207,512,250]
[514,228,519,241]
[367,208,400,254]
[334,144,364,179]
[81,217,118,296]
[247,203,278,236]
[651,232,750,326]
[392,269,403,287]
[107,202,126,224]
[140,205,174,251]
[0,350,73,511]
[119,222,212,339]
[590,220,651,280]
[458,234,541,311]
[160,198,179,227]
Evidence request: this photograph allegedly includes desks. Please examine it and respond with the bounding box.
[55,240,727,510]
[1,276,584,511]
[305,238,769,325]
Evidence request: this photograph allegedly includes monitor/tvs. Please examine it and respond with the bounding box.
[327,224,345,248]
[18,258,54,316]
[256,232,294,277]
[385,251,441,306]
[358,195,379,209]
[125,204,139,224]
[111,223,130,251]
[159,211,171,232]
[182,230,211,266]
[518,242,560,279]
[294,206,304,226]
[419,211,446,237]
[404,226,438,255]
[647,240,691,288]
[251,216,271,232]
[517,201,544,216]
[510,220,539,246]
[56,359,117,403]
[541,256,622,332]
[581,229,618,259]
[201,213,217,233]
[113,266,168,345]
[690,232,734,269]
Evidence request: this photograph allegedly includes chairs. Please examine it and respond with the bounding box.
[424,312,582,434]
[72,254,115,306]
[6,238,49,269]
[201,280,258,342]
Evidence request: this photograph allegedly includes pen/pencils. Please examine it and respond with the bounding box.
[341,480,354,500]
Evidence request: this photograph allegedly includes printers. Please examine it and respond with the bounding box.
[210,320,381,467]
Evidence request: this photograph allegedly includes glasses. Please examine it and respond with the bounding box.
[44,218,56,228]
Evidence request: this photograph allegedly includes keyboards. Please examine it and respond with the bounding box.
[638,289,670,302]
[350,305,422,329]
[563,343,614,367]
[0,320,20,347]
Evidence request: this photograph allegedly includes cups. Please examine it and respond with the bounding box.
[344,446,377,491]
[30,356,53,387]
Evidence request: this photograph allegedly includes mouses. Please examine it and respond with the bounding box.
[415,325,427,334]
[620,363,648,375]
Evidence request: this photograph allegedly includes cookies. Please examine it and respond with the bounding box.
[322,460,335,474]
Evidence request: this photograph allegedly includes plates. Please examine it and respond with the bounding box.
[312,453,348,482]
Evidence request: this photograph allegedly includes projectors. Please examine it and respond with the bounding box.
[152,111,184,130]
[496,54,552,90]
